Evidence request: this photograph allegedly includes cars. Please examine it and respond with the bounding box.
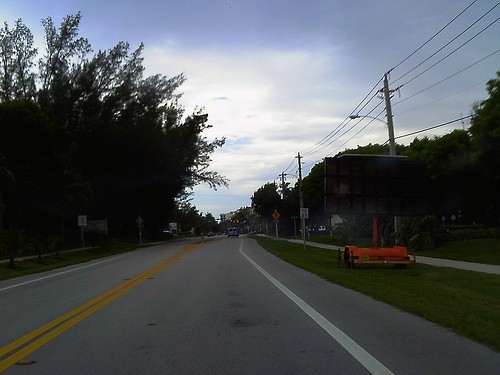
[208,231,214,237]
[310,226,325,231]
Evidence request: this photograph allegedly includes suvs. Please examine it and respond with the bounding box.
[227,227,240,238]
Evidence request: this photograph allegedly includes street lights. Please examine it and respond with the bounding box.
[279,174,306,239]
[349,112,404,247]
